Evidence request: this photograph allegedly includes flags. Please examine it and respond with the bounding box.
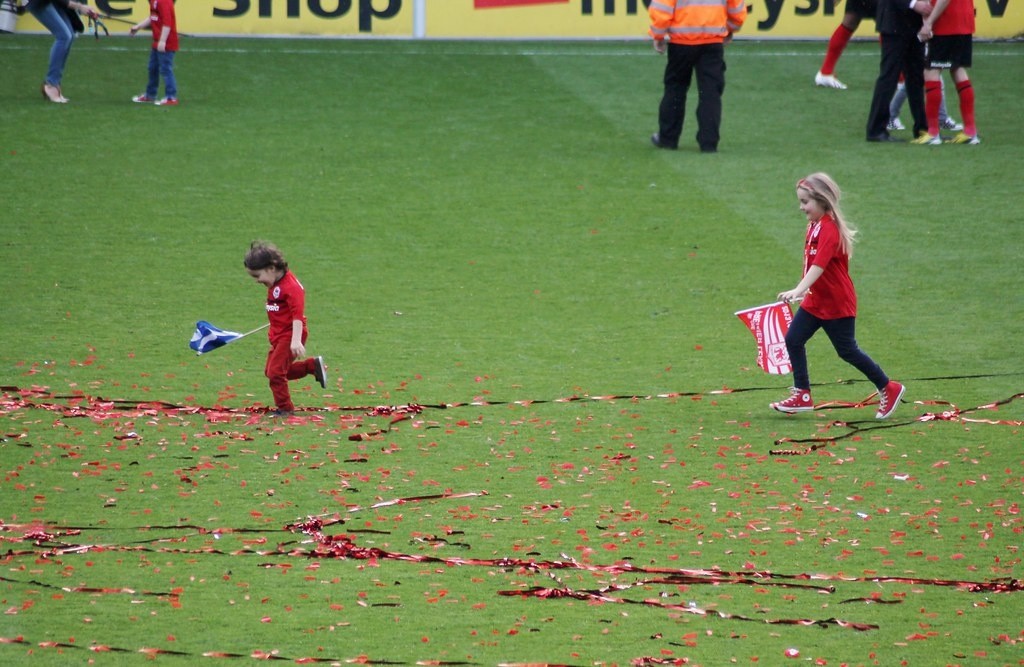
[188,320,243,355]
[735,302,795,375]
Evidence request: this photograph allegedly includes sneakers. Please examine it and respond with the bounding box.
[939,116,963,131]
[768,386,815,412]
[886,118,905,130]
[132,95,155,103]
[814,71,847,90]
[910,130,942,145]
[942,131,980,145]
[875,379,906,419]
[154,97,179,106]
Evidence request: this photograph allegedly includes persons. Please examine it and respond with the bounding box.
[647,0,748,153]
[814,0,981,146]
[128,0,180,105]
[243,239,327,417]
[767,172,906,419]
[21,0,97,103]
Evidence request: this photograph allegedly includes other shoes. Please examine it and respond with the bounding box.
[699,142,717,152]
[866,131,897,142]
[651,132,679,150]
[313,356,327,389]
[41,81,70,104]
